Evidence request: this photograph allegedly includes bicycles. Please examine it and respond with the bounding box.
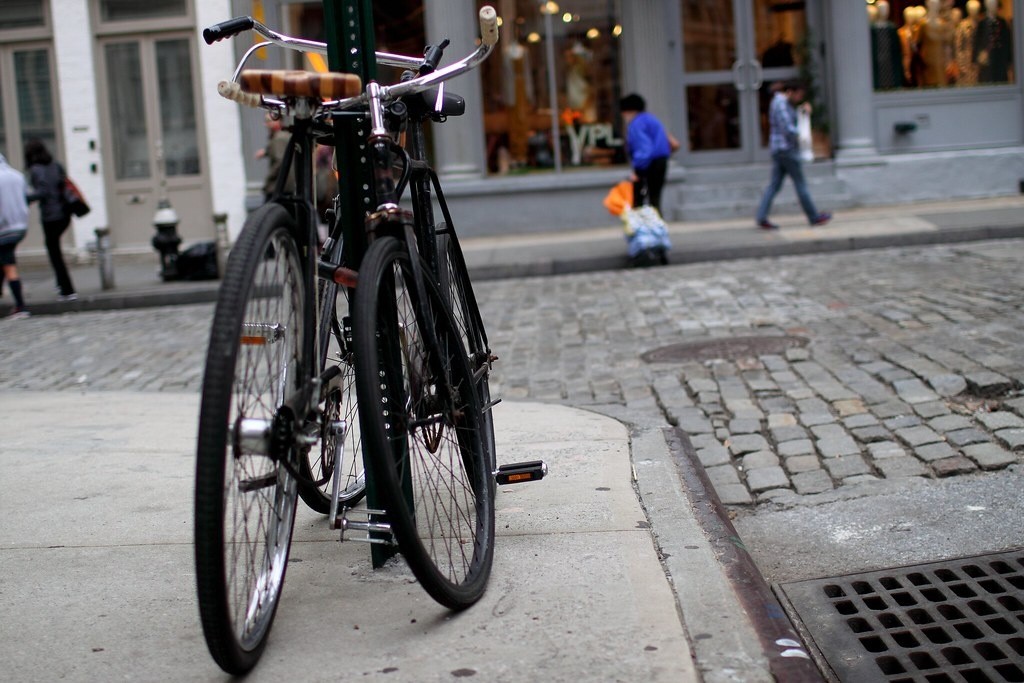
[190,4,549,677]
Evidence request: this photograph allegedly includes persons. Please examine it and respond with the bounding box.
[619,92,670,221]
[24,140,79,302]
[488,129,515,178]
[752,77,832,231]
[870,0,1012,91]
[0,151,31,324]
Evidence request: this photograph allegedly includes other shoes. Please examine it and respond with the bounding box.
[755,220,779,229]
[56,293,78,301]
[812,212,832,225]
[4,308,30,321]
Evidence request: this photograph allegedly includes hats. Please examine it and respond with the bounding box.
[264,114,280,130]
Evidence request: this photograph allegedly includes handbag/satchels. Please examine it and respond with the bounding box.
[663,132,679,153]
[977,51,990,65]
[796,107,814,164]
[56,164,90,217]
[602,181,633,218]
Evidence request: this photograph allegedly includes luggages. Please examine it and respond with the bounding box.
[621,177,671,266]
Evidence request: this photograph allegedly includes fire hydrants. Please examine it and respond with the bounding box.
[149,208,182,281]
